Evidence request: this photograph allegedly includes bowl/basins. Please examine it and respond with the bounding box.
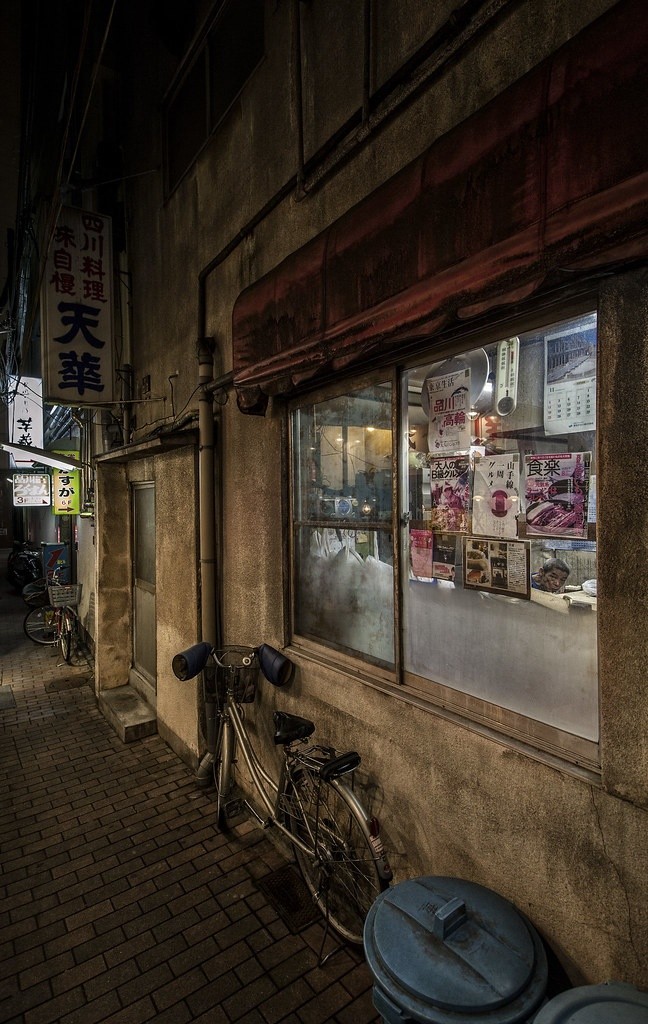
[548,478,589,504]
[468,549,486,564]
[527,499,585,535]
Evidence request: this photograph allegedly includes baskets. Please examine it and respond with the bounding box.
[201,644,260,704]
[49,583,81,608]
[23,578,51,608]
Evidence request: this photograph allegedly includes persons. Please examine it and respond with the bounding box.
[530,558,570,593]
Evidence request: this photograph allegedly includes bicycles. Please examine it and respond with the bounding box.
[4,537,43,590]
[21,564,85,667]
[172,640,396,970]
